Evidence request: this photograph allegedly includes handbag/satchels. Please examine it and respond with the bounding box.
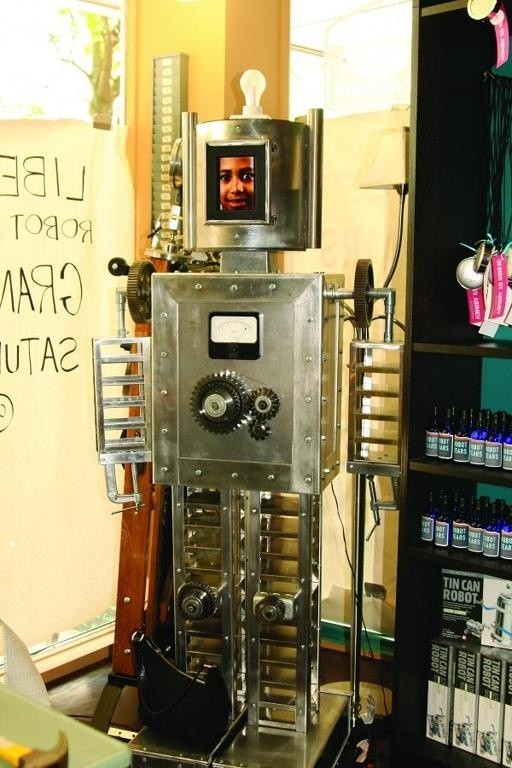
[130,631,230,758]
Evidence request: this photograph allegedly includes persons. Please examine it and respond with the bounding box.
[218,156,255,210]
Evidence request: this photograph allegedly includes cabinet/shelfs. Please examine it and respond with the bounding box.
[392,0,510,768]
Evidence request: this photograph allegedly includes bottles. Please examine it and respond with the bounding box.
[419,489,512,562]
[424,404,512,472]
